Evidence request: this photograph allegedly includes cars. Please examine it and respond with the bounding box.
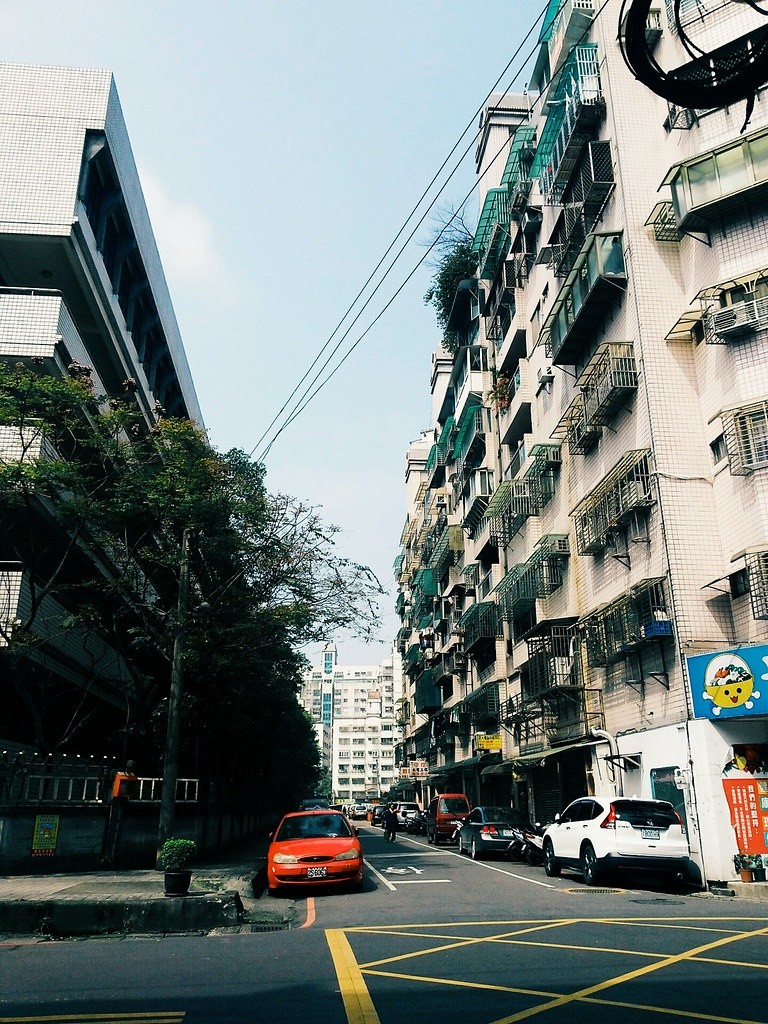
[458,805,536,859]
[347,804,368,818]
[267,808,364,890]
[368,805,388,826]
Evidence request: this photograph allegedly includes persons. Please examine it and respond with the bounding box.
[383,803,401,844]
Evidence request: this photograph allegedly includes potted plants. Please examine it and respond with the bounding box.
[162,838,198,898]
[733,851,768,883]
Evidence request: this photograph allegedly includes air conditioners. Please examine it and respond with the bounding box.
[545,450,560,461]
[572,75,599,113]
[537,366,555,383]
[513,482,530,497]
[519,141,537,161]
[425,648,433,660]
[619,481,645,510]
[519,211,542,232]
[710,300,755,339]
[550,538,570,553]
[546,657,571,688]
[645,18,657,30]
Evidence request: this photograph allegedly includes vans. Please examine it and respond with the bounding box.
[425,794,471,846]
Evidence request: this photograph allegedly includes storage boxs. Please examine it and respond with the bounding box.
[644,619,672,638]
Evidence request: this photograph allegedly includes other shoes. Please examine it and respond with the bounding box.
[393,841,396,843]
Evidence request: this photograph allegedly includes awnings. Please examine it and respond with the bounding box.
[422,752,513,787]
[510,739,609,771]
[390,780,415,790]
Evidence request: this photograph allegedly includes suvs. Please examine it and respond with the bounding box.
[541,794,692,887]
[381,800,422,832]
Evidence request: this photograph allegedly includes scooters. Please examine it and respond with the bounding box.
[507,812,561,866]
